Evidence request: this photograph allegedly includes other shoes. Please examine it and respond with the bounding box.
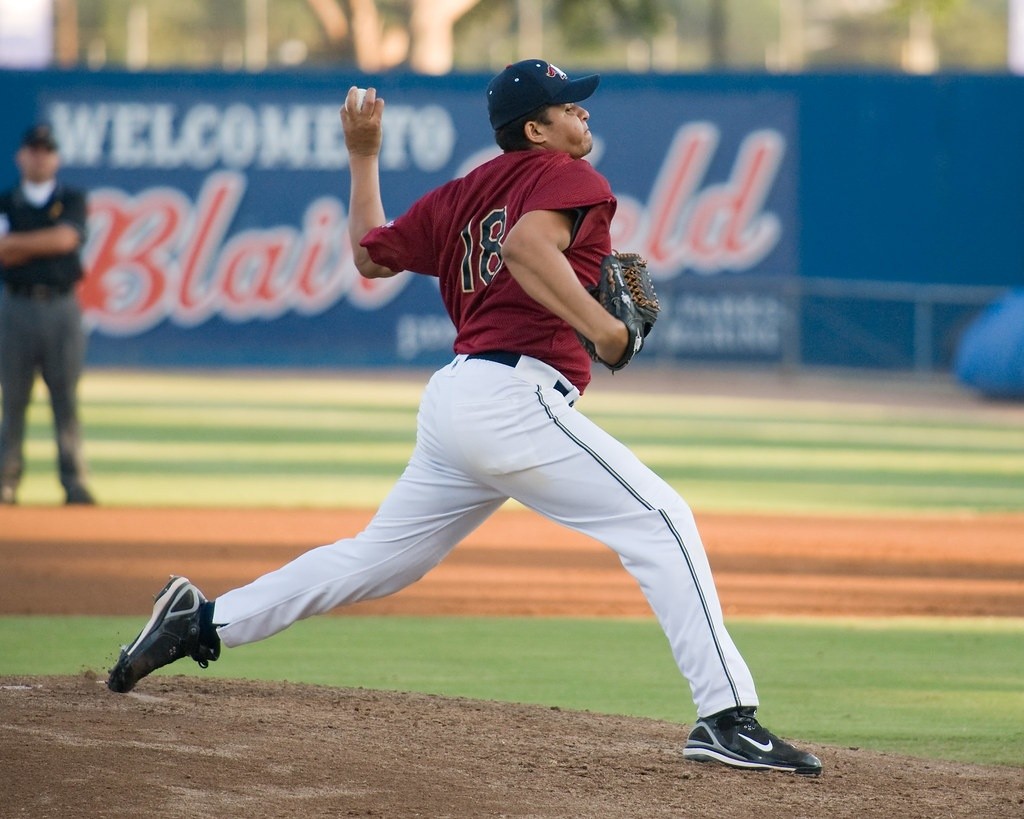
[63,488,100,508]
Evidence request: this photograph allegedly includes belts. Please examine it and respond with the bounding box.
[469,349,574,406]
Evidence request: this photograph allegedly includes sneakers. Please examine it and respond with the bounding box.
[103,577,222,694]
[684,708,824,776]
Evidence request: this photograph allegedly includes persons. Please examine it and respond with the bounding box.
[1,124,95,504]
[110,60,824,778]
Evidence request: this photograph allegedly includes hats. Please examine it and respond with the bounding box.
[483,58,600,130]
[20,122,65,154]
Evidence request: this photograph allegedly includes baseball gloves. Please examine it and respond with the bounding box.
[567,247,666,375]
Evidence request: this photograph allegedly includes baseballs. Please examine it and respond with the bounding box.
[343,86,374,117]
